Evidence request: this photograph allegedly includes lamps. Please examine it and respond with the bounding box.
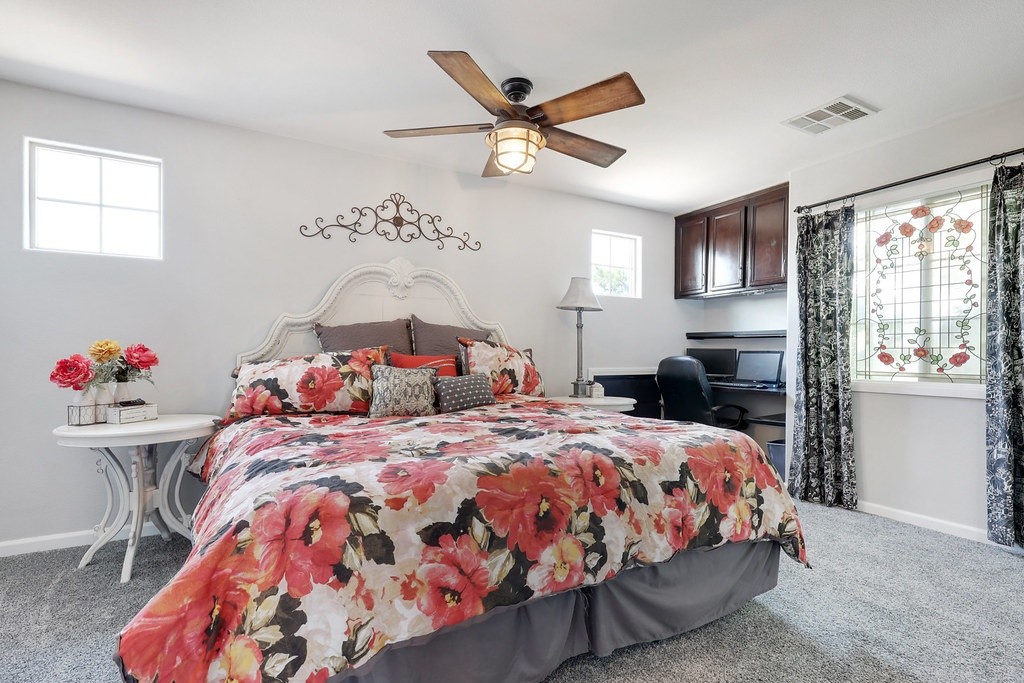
[555,277,604,397]
[485,121,547,174]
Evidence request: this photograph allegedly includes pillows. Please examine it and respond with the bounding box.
[368,365,440,418]
[411,313,491,375]
[455,336,546,397]
[314,319,413,355]
[390,353,459,377]
[431,372,499,414]
[213,345,390,430]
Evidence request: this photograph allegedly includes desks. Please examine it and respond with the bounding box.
[52,414,223,583]
[552,396,637,411]
[708,381,786,393]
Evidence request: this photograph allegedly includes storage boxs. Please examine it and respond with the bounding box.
[108,403,158,425]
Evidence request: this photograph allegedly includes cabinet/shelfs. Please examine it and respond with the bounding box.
[674,181,789,299]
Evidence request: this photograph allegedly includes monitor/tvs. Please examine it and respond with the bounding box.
[687,348,737,382]
[735,350,785,388]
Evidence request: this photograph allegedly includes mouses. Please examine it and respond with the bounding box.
[756,383,769,389]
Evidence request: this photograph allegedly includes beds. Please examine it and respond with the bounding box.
[113,257,813,683]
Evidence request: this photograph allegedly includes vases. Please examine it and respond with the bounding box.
[73,390,95,405]
[95,383,114,404]
[113,383,129,403]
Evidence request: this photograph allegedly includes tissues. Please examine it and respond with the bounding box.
[589,382,604,398]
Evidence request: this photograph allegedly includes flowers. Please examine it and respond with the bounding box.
[50,339,160,396]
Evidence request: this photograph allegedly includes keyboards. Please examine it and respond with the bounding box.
[708,382,757,387]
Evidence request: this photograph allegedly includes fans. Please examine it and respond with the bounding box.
[383,51,646,177]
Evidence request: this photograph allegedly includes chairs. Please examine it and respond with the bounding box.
[655,356,748,430]
